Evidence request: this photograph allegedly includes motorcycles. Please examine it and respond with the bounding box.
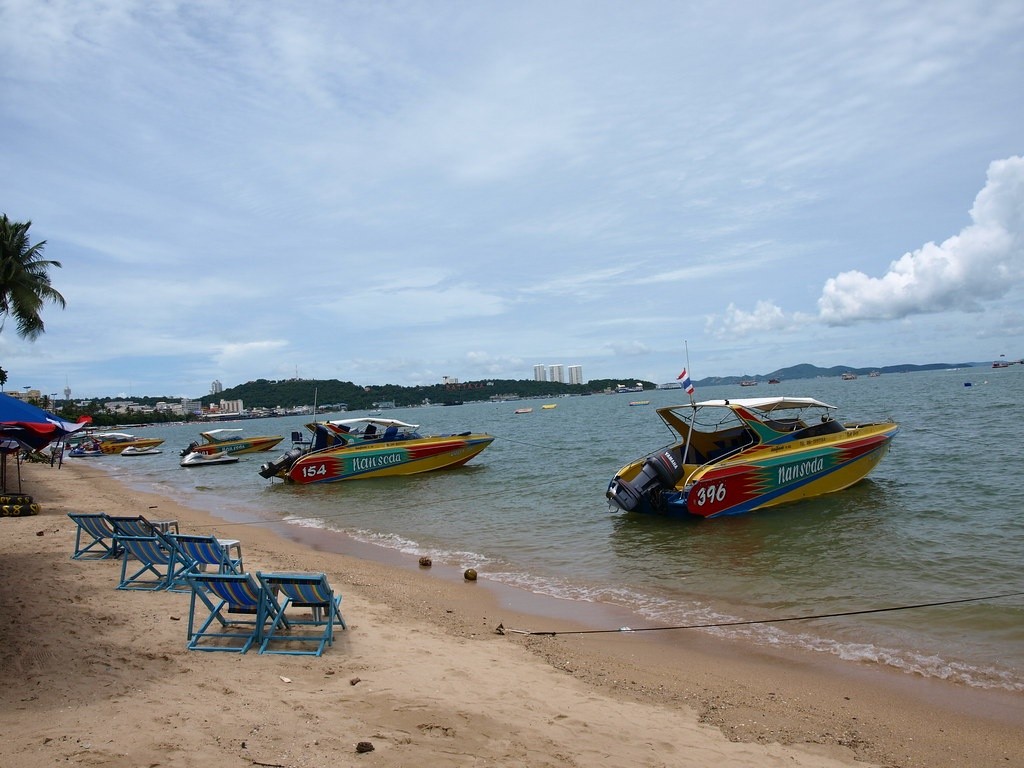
[68,445,104,457]
[121,445,163,455]
[180,452,240,466]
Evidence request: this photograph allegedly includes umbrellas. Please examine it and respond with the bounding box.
[0,392,88,493]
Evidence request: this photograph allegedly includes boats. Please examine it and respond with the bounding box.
[542,404,558,410]
[368,412,382,416]
[843,372,858,380]
[991,361,1014,368]
[868,371,881,377]
[768,379,780,384]
[259,417,497,484]
[76,433,166,454]
[605,395,901,521]
[627,401,649,405]
[740,381,758,387]
[179,428,284,457]
[514,407,533,413]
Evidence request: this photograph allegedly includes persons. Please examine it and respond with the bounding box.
[86,444,91,451]
[92,441,102,451]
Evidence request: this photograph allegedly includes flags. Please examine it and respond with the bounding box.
[677,369,694,394]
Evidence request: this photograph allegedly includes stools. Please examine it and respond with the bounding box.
[198,539,244,573]
[148,518,179,535]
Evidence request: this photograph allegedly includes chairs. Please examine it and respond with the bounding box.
[254,569,348,657]
[112,533,196,593]
[164,529,242,599]
[181,570,286,655]
[104,514,175,559]
[65,510,123,560]
